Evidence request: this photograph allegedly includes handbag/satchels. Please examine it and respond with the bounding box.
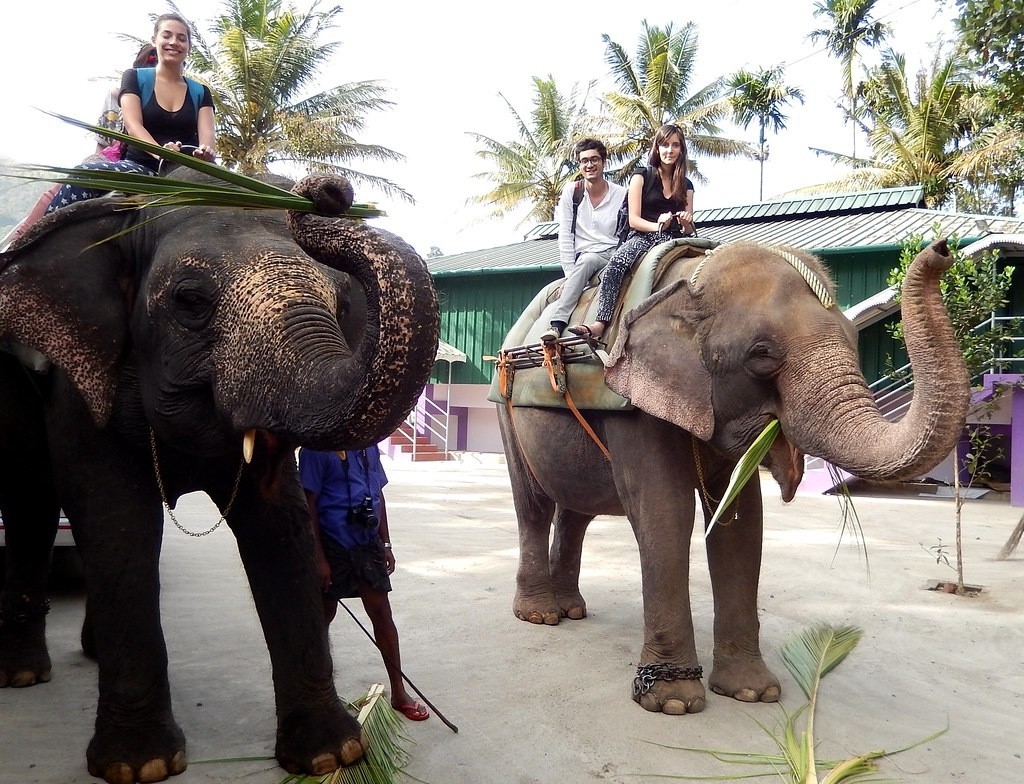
[98,138,126,162]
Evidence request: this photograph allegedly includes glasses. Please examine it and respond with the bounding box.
[579,156,602,165]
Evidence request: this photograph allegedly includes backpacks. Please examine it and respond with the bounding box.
[614,167,658,249]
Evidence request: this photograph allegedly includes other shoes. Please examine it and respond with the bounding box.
[539,326,560,341]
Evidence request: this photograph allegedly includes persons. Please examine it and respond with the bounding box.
[13,13,218,242]
[540,124,695,342]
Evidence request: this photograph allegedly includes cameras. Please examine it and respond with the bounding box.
[347,497,379,529]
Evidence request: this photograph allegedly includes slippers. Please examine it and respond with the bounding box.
[392,698,429,721]
[568,324,601,348]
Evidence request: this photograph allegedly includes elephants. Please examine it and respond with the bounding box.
[0,172,444,782]
[488,237,970,715]
